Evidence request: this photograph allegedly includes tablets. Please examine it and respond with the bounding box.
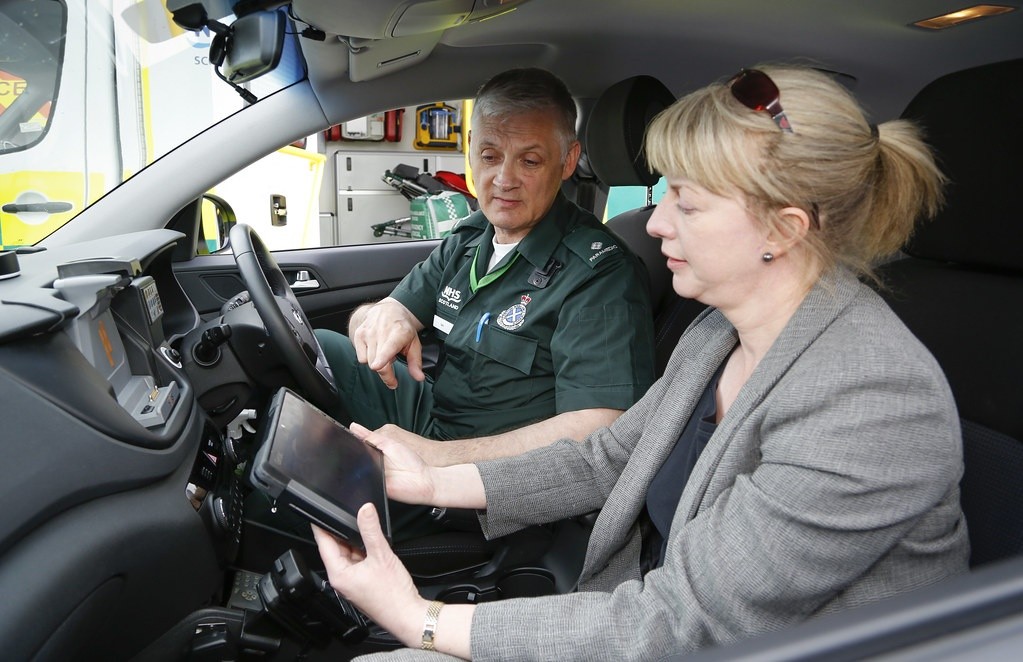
[251,387,393,554]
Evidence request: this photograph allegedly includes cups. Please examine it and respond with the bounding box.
[430,111,450,140]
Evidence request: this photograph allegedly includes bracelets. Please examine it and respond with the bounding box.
[422,597,444,650]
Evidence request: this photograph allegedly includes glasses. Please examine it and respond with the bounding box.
[722,67,820,232]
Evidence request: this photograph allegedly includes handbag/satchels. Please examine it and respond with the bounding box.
[410,190,471,239]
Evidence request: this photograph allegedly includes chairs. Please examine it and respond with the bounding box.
[588,74,708,382]
[859,55,1023,568]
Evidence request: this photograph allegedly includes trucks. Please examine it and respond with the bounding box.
[0,0,676,262]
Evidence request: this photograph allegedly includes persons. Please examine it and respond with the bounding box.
[311,61,973,662]
[312,67,657,436]
